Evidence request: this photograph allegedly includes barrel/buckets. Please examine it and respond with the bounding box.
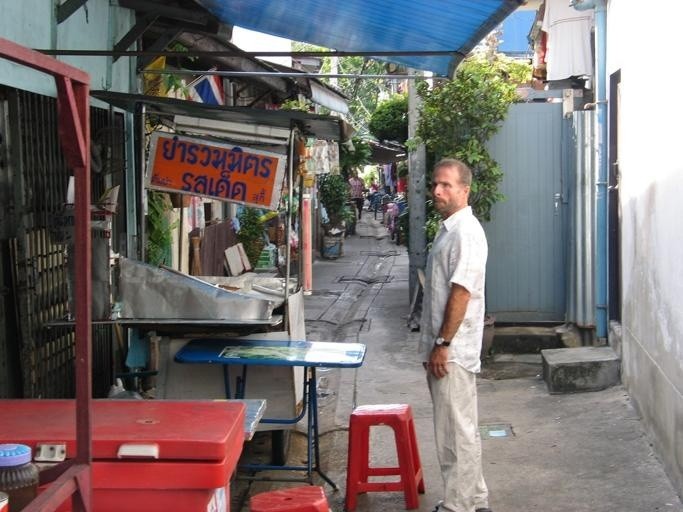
[322,236,342,259]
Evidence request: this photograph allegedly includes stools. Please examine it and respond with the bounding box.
[249,404,426,511]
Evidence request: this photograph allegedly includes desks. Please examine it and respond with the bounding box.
[170,336,367,492]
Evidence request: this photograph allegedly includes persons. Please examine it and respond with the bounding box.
[396,168,408,198]
[416,159,493,512]
[347,168,365,220]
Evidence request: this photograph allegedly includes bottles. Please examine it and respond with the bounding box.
[0,443,38,512]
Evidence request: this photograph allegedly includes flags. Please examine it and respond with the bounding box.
[141,56,168,100]
[185,67,226,108]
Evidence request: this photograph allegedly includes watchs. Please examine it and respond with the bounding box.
[432,336,450,347]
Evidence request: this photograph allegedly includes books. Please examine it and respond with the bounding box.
[235,242,251,272]
[224,241,244,277]
[251,274,297,298]
